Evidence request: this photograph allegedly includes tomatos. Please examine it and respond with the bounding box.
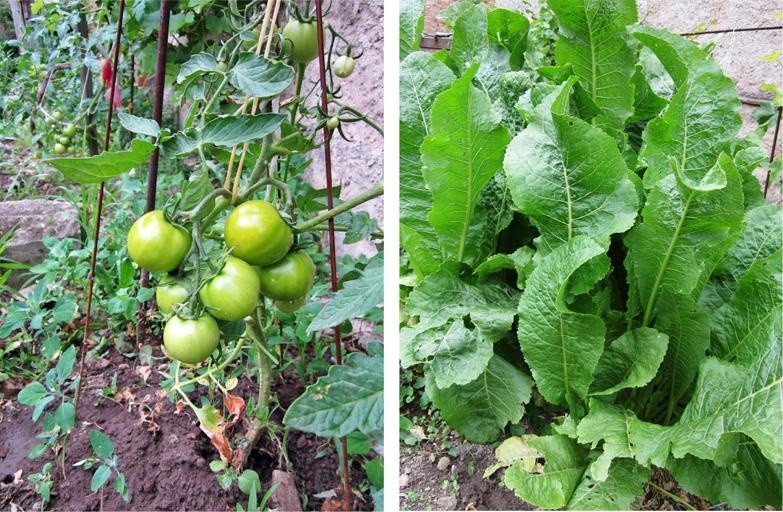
[30,13,356,365]
